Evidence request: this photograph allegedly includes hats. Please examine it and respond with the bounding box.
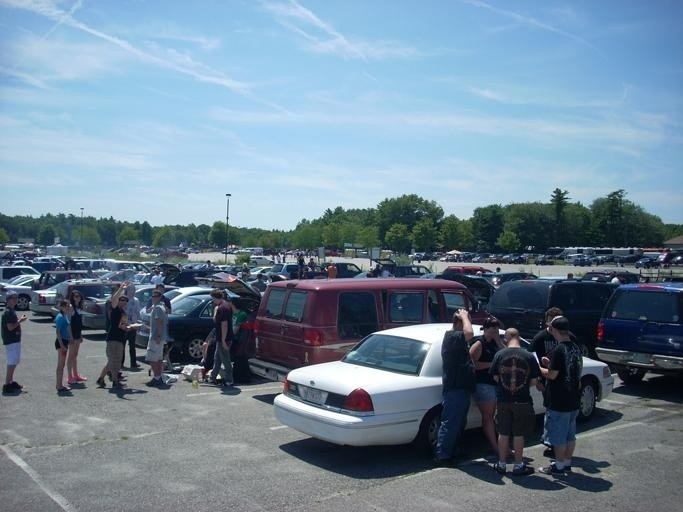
[545,316,567,329]
[479,316,495,330]
[6,290,17,298]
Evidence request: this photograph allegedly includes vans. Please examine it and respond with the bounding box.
[490,280,619,358]
[248,278,503,381]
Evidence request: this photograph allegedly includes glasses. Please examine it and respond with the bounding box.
[120,300,129,302]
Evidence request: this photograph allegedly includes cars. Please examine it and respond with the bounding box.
[134,294,261,364]
[594,283,682,383]
[272,322,613,451]
[107,240,305,255]
[411,246,681,272]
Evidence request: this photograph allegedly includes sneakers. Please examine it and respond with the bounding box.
[433,456,455,467]
[56,385,71,392]
[12,382,23,388]
[96,377,106,386]
[217,381,233,386]
[513,466,534,475]
[3,385,17,392]
[67,377,82,384]
[452,455,465,463]
[563,465,571,472]
[538,462,565,476]
[113,382,127,388]
[72,375,87,381]
[201,378,216,383]
[150,377,169,389]
[491,463,506,473]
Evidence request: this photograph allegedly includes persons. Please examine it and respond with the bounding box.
[53,248,340,395]
[433,306,475,467]
[1,289,29,395]
[529,308,580,455]
[537,316,584,477]
[0,248,43,267]
[365,259,649,287]
[469,315,519,459]
[486,328,542,476]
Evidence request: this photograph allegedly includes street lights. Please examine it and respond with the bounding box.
[225,193,232,266]
[79,207,84,254]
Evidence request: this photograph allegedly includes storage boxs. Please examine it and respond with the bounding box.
[181,365,207,381]
